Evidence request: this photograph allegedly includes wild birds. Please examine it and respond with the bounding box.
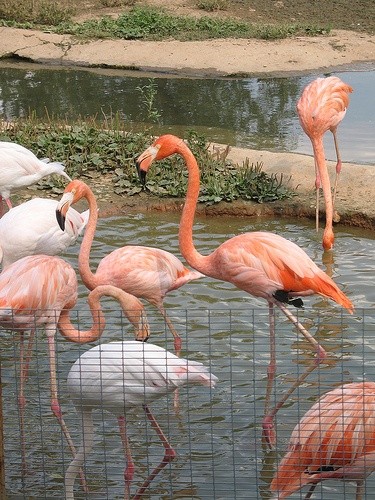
[64,339,216,500]
[55,178,207,415]
[1,253,151,499]
[270,380,375,500]
[0,197,100,382]
[136,134,355,451]
[296,75,353,253]
[0,141,73,219]
